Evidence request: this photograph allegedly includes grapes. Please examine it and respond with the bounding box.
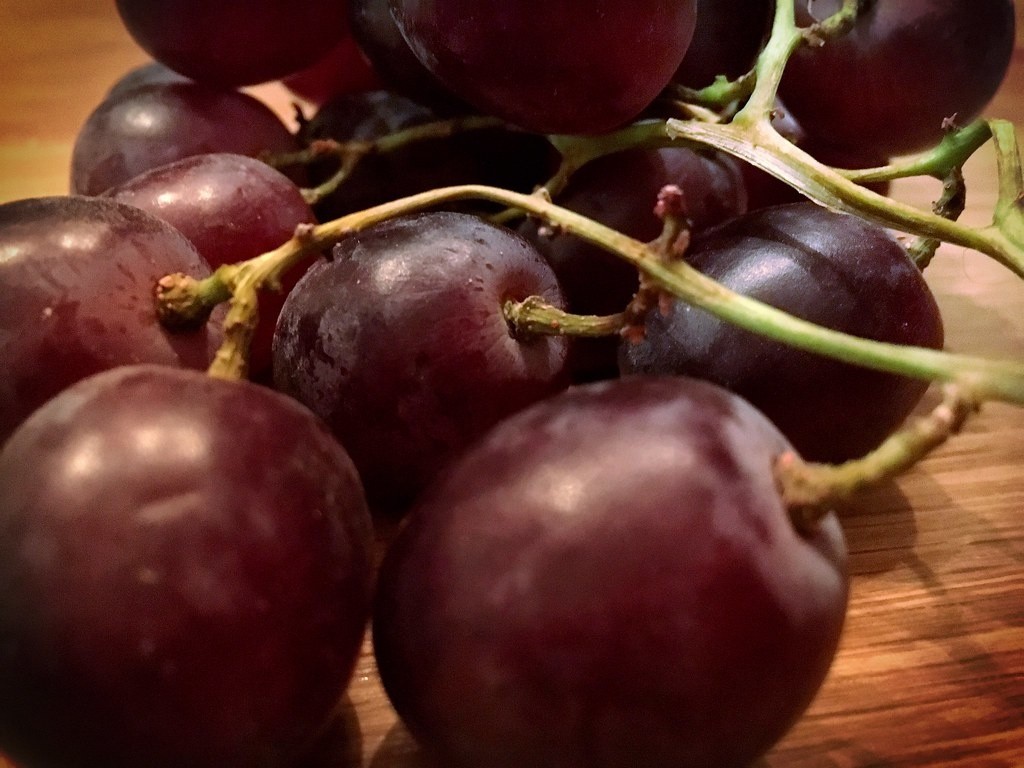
[0,0,1015,768]
